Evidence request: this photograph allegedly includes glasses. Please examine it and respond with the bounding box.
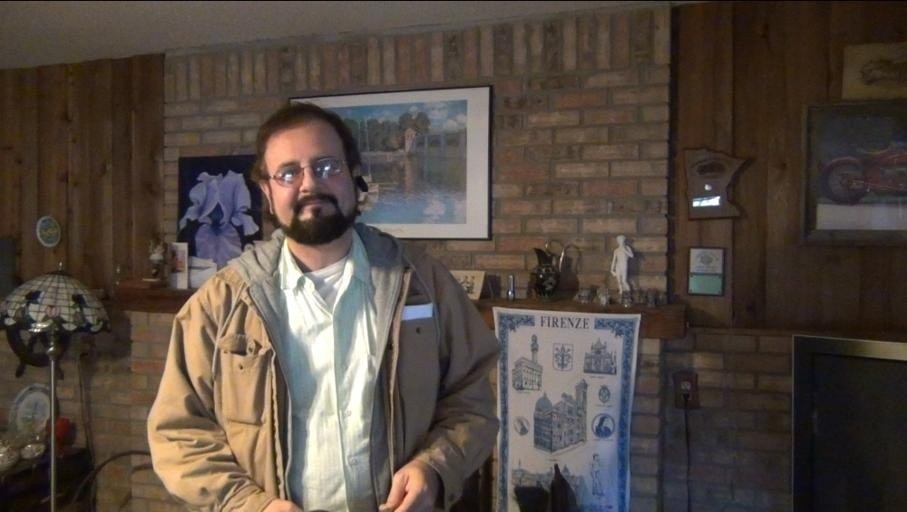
[267,155,354,189]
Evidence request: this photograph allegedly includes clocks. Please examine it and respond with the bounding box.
[9,384,58,439]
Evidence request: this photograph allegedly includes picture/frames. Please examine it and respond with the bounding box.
[800,101,907,248]
[287,85,492,241]
[840,39,907,103]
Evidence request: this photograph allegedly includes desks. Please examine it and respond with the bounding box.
[0,442,94,512]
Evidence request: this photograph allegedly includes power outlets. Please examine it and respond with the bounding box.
[672,370,700,410]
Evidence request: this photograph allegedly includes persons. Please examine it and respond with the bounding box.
[610,234,634,294]
[146,100,504,512]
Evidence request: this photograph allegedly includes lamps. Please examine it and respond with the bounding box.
[0,259,110,512]
